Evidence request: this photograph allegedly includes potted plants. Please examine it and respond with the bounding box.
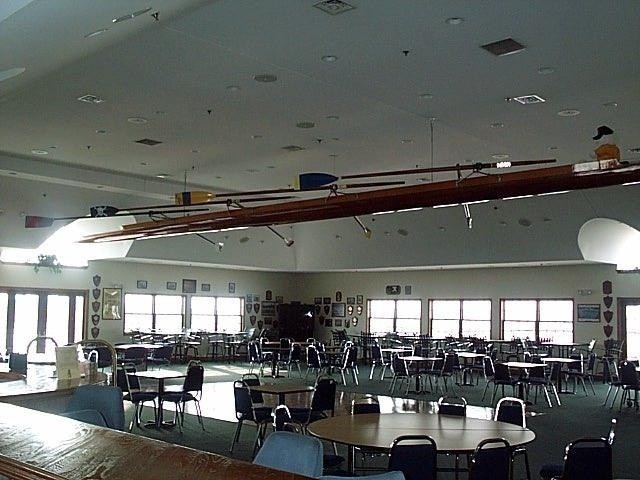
[167,282,176,290]
[577,303,600,323]
[102,287,122,320]
[246,290,283,325]
[314,295,363,327]
[182,279,197,293]
[201,284,210,291]
[229,283,235,293]
[136,281,147,289]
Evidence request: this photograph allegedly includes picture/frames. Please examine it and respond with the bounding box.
[201,284,210,291]
[577,303,600,323]
[136,281,147,289]
[229,283,235,293]
[182,279,197,293]
[102,287,122,320]
[167,282,176,290]
[246,290,283,325]
[314,295,363,327]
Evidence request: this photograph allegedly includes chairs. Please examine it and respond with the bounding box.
[368,329,639,412]
[68,339,206,435]
[130,328,375,387]
[229,373,338,458]
[253,395,613,478]
